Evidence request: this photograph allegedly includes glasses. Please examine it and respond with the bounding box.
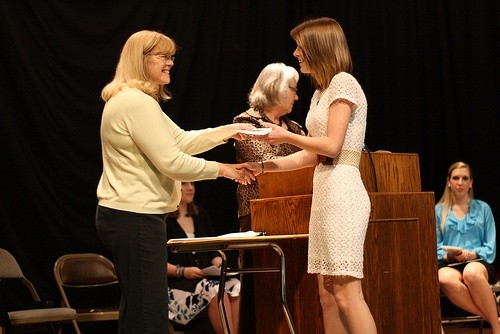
[145,53,175,62]
[289,86,298,94]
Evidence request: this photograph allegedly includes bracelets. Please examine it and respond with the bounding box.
[182,267,185,278]
[176,266,180,277]
[261,162,265,174]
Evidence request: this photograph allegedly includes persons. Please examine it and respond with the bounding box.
[235,62,310,334]
[430,162,500,334]
[94,28,258,333]
[235,16,379,334]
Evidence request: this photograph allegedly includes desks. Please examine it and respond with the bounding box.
[167,233,308,334]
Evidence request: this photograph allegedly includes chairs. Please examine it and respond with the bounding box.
[54,253,120,334]
[440,282,500,334]
[0,247,77,334]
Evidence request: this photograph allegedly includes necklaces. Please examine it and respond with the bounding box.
[277,117,283,126]
